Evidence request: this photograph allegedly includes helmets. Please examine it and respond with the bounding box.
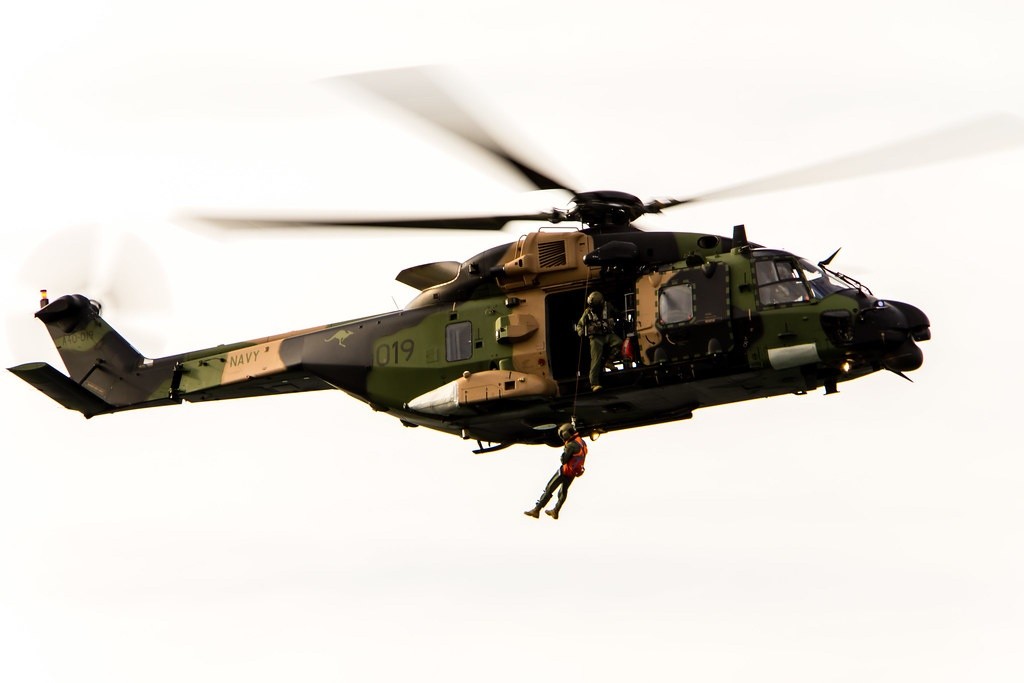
[587,291,603,305]
[558,423,574,438]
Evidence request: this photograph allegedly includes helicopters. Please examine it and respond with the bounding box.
[4,64,1022,453]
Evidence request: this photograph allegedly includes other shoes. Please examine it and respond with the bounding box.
[524,510,539,519]
[545,510,558,519]
[591,384,602,392]
[604,361,618,371]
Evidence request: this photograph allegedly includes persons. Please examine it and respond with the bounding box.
[771,267,804,302]
[524,423,587,520]
[576,292,622,392]
